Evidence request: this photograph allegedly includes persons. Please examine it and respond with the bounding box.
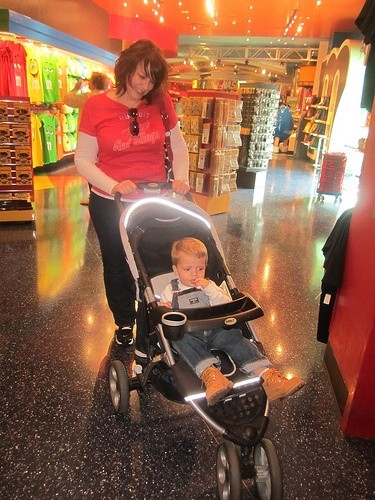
[64,72,110,205]
[157,238,306,406]
[74,39,190,358]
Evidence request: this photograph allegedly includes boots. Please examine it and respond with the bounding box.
[199,364,234,407]
[261,367,305,402]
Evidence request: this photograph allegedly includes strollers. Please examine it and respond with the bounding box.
[104,180,285,500]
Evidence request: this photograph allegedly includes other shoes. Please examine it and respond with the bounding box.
[79,197,89,205]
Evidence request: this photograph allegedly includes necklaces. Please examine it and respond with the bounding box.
[122,94,136,107]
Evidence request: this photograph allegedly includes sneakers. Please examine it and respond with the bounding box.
[116,324,133,347]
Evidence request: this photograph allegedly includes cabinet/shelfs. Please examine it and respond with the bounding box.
[300,96,331,160]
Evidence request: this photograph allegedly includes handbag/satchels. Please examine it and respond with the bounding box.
[133,279,163,373]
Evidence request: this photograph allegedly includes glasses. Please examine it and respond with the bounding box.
[128,108,140,136]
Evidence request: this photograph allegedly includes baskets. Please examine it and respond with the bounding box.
[318,152,347,193]
